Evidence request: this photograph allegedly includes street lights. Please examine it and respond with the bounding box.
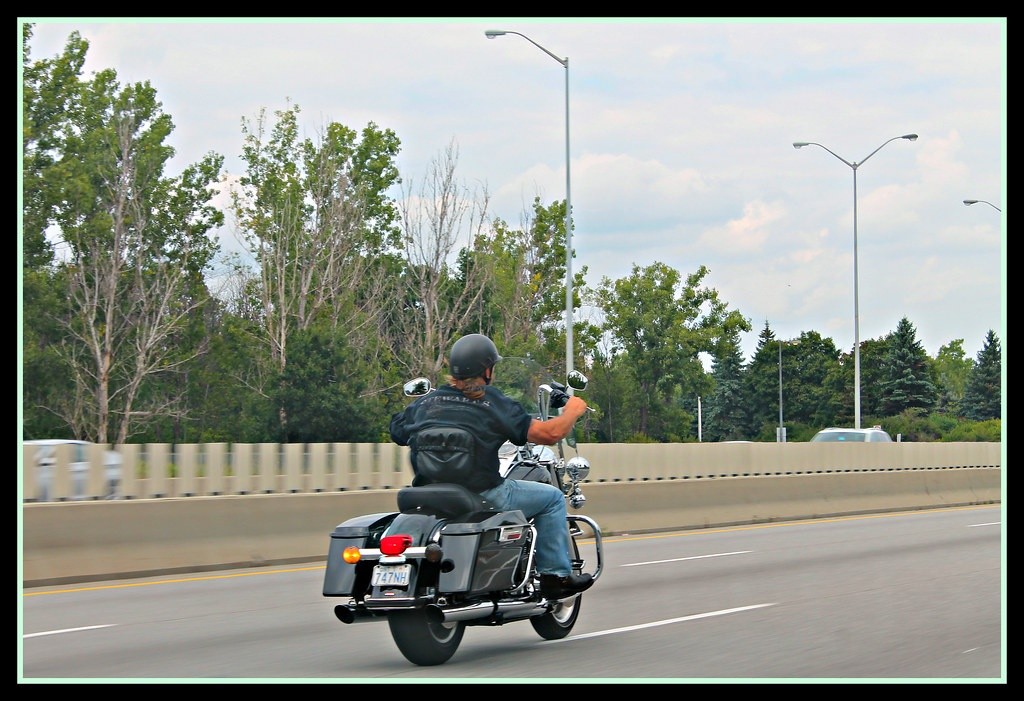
[793,133,920,430]
[482,23,575,394]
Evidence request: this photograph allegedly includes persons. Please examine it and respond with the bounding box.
[389,334,593,599]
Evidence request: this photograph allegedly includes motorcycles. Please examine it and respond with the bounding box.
[320,370,606,666]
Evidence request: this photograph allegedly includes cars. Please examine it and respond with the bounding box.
[809,427,897,444]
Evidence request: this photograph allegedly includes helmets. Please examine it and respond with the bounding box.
[449,334,502,379]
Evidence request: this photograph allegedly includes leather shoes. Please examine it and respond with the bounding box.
[540,573,592,601]
